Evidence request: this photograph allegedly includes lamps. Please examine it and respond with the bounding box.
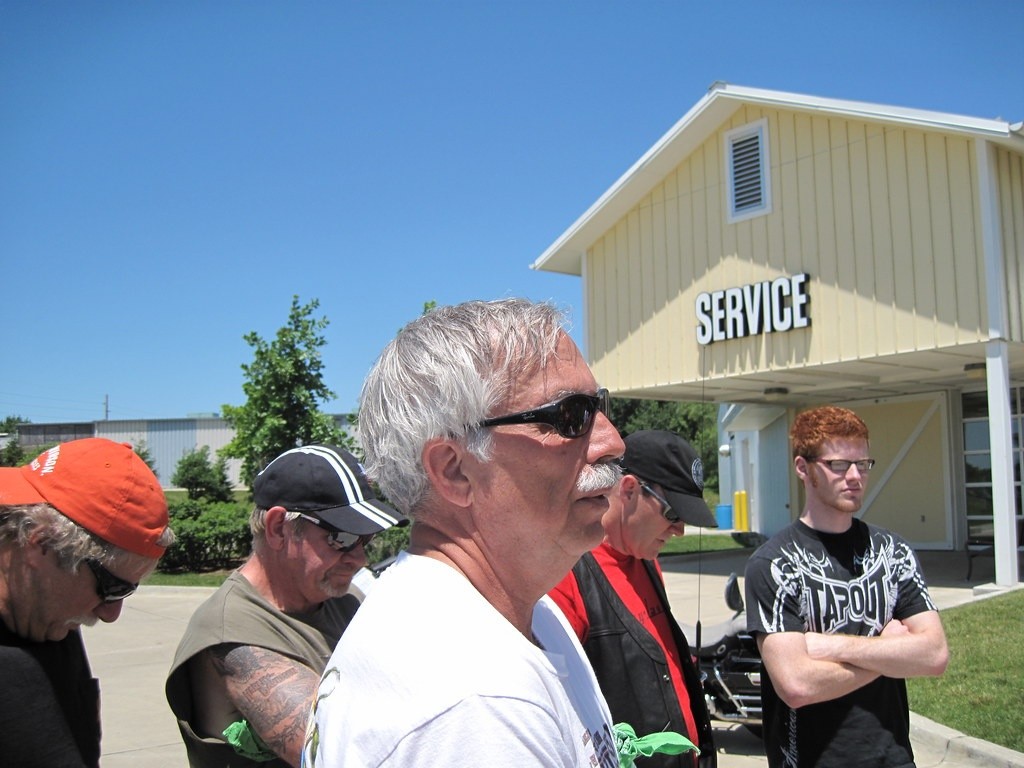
[963,362,988,380]
[765,388,789,403]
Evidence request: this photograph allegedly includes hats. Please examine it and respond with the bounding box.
[0,437,169,560]
[614,431,719,529]
[256,444,410,535]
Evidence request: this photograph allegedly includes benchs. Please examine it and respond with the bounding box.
[965,518,1024,581]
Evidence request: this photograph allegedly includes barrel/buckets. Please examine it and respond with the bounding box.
[715,505,732,529]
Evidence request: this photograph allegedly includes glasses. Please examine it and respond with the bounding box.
[450,388,610,438]
[84,558,140,603]
[638,481,681,523]
[297,513,376,552]
[806,457,875,472]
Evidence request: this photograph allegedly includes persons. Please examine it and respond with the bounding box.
[0,438,175,768]
[547,429,720,768]
[166,446,411,768]
[298,297,626,768]
[745,406,948,768]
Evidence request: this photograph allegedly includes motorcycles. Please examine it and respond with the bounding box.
[676,569,766,742]
